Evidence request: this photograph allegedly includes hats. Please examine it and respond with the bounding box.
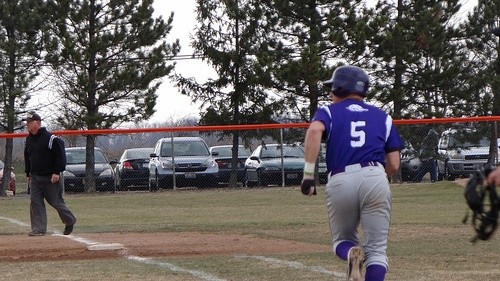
[462,163,500,243]
[21,114,41,121]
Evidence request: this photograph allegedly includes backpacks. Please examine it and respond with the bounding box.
[49,134,67,172]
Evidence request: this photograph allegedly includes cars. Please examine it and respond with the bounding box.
[63,146,118,192]
[317,143,334,182]
[0,160,16,194]
[211,145,250,175]
[243,143,307,186]
[400,146,424,180]
[112,147,155,190]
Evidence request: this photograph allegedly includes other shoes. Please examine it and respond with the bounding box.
[63,216,76,235]
[28,230,46,236]
[347,245,366,281]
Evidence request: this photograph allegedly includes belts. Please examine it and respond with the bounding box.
[325,159,379,184]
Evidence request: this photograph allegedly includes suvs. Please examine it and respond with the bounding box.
[436,128,500,181]
[147,136,221,190]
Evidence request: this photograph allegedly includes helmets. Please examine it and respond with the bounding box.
[323,66,370,98]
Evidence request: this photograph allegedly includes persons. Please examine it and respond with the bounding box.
[301,65,404,281]
[414,124,444,183]
[22,112,76,236]
[488,166,500,187]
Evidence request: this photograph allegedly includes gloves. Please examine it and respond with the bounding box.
[300,170,318,196]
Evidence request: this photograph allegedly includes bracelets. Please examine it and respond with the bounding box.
[303,171,314,175]
